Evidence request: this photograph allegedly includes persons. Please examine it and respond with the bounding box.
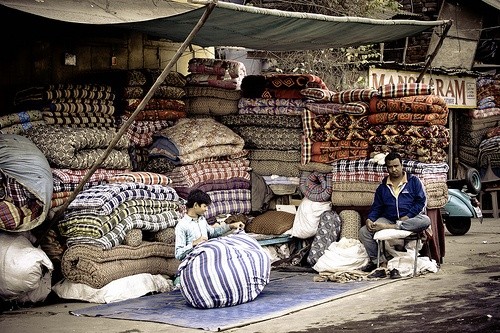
[358,152,430,272]
[175,189,245,260]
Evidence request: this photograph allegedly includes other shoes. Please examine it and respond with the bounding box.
[367,269,387,279]
[389,268,401,279]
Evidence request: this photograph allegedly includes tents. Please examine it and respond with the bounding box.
[0,0,455,248]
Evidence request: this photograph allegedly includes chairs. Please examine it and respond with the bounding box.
[373,199,430,277]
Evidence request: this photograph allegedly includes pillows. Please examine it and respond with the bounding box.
[245,210,295,234]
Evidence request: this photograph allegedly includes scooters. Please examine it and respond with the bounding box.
[440,168,483,235]
